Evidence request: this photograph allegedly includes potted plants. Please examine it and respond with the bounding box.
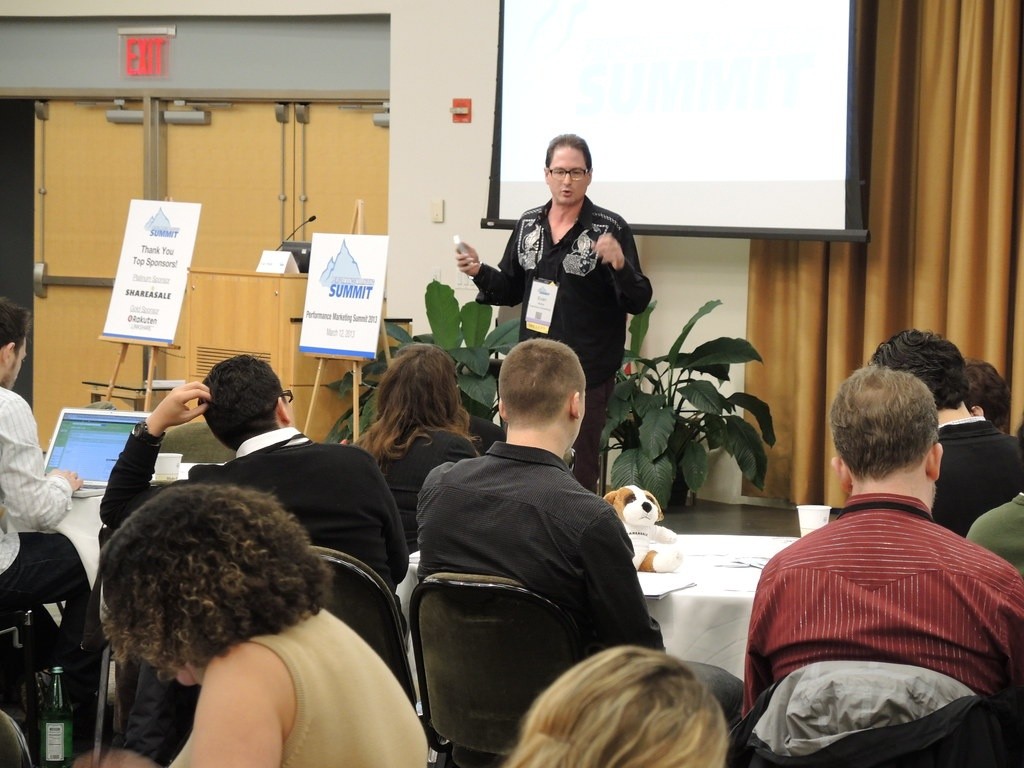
[597,301,776,513]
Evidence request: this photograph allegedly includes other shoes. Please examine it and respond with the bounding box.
[74,698,113,736]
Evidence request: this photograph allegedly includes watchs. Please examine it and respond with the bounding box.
[131,421,166,446]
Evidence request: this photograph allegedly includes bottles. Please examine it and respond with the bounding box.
[39,667,74,768]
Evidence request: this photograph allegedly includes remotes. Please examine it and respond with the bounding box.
[452,235,473,266]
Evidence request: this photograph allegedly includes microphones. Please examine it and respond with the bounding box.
[277,216,316,251]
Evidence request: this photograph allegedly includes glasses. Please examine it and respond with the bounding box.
[273,390,293,411]
[547,167,589,181]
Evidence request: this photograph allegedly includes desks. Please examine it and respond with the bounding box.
[48,487,804,716]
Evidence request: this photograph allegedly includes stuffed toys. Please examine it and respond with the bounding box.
[599,484,685,574]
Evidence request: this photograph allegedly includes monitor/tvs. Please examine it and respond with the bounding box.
[282,242,312,273]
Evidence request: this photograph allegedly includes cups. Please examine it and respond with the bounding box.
[796,505,832,538]
[155,453,183,482]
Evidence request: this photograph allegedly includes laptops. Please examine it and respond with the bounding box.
[43,406,155,499]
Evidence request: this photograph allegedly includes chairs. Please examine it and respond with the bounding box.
[0,547,593,767]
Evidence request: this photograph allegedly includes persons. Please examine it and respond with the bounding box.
[99,481,428,768]
[742,361,1024,721]
[415,337,744,726]
[965,490,1024,580]
[0,296,84,652]
[338,342,480,555]
[454,133,653,495]
[963,357,1009,434]
[869,328,1024,539]
[497,644,729,768]
[98,354,409,638]
